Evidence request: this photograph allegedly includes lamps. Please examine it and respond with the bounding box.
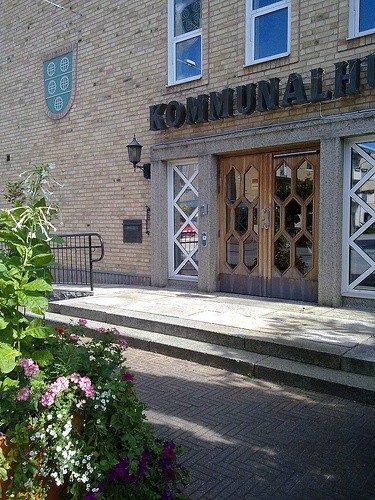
[126,132,150,180]
